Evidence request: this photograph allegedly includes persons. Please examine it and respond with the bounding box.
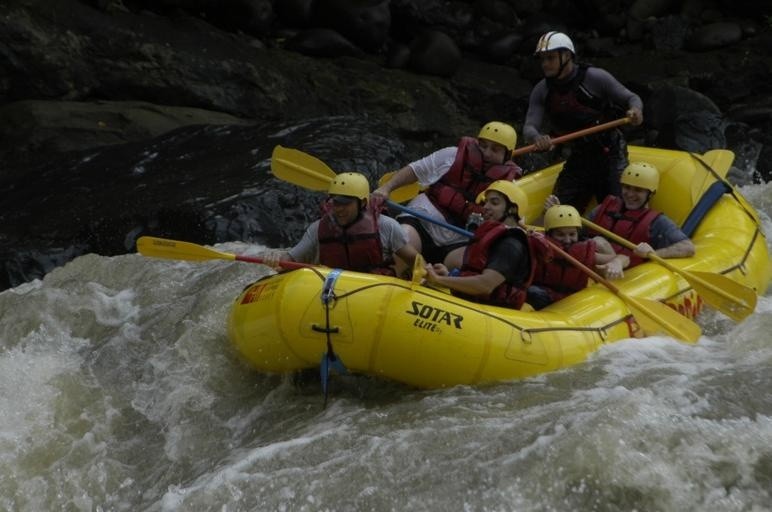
[542,161,695,271]
[522,31,644,217]
[423,180,537,310]
[372,121,517,279]
[518,205,631,310]
[262,171,435,286]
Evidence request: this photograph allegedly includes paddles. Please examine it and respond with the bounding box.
[270,145,475,238]
[410,252,429,285]
[519,221,701,348]
[677,147,735,244]
[377,111,638,204]
[136,236,314,268]
[581,216,757,322]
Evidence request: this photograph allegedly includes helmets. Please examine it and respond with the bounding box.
[328,171,372,210]
[621,162,661,194]
[485,179,528,219]
[534,30,576,56]
[544,204,582,232]
[478,120,517,151]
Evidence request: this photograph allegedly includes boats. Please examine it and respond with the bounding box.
[222,141,769,398]
